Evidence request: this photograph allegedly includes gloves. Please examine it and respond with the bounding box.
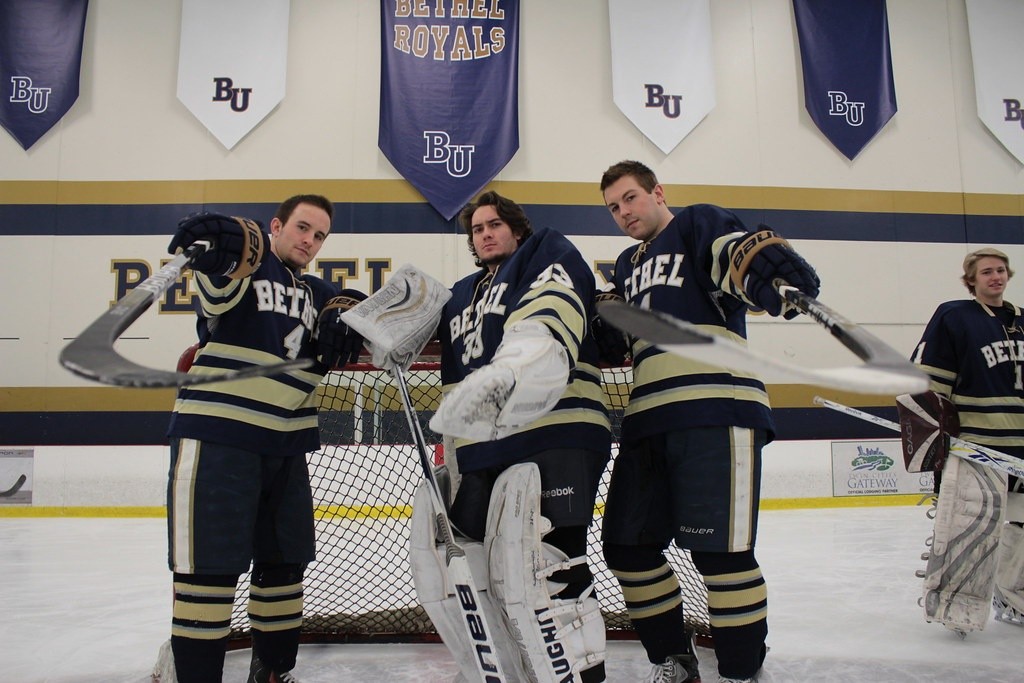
[590,290,628,365]
[731,225,820,322]
[168,211,270,278]
[307,290,370,369]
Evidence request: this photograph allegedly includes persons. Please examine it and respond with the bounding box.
[589,161,821,683]
[167,194,369,683]
[372,190,610,683]
[896,248,1024,640]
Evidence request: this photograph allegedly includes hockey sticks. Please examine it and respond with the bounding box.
[599,276,931,395]
[813,395,1024,480]
[59,240,313,386]
[387,364,509,682]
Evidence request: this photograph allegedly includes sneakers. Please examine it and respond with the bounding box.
[647,630,702,683]
[247,651,299,683]
